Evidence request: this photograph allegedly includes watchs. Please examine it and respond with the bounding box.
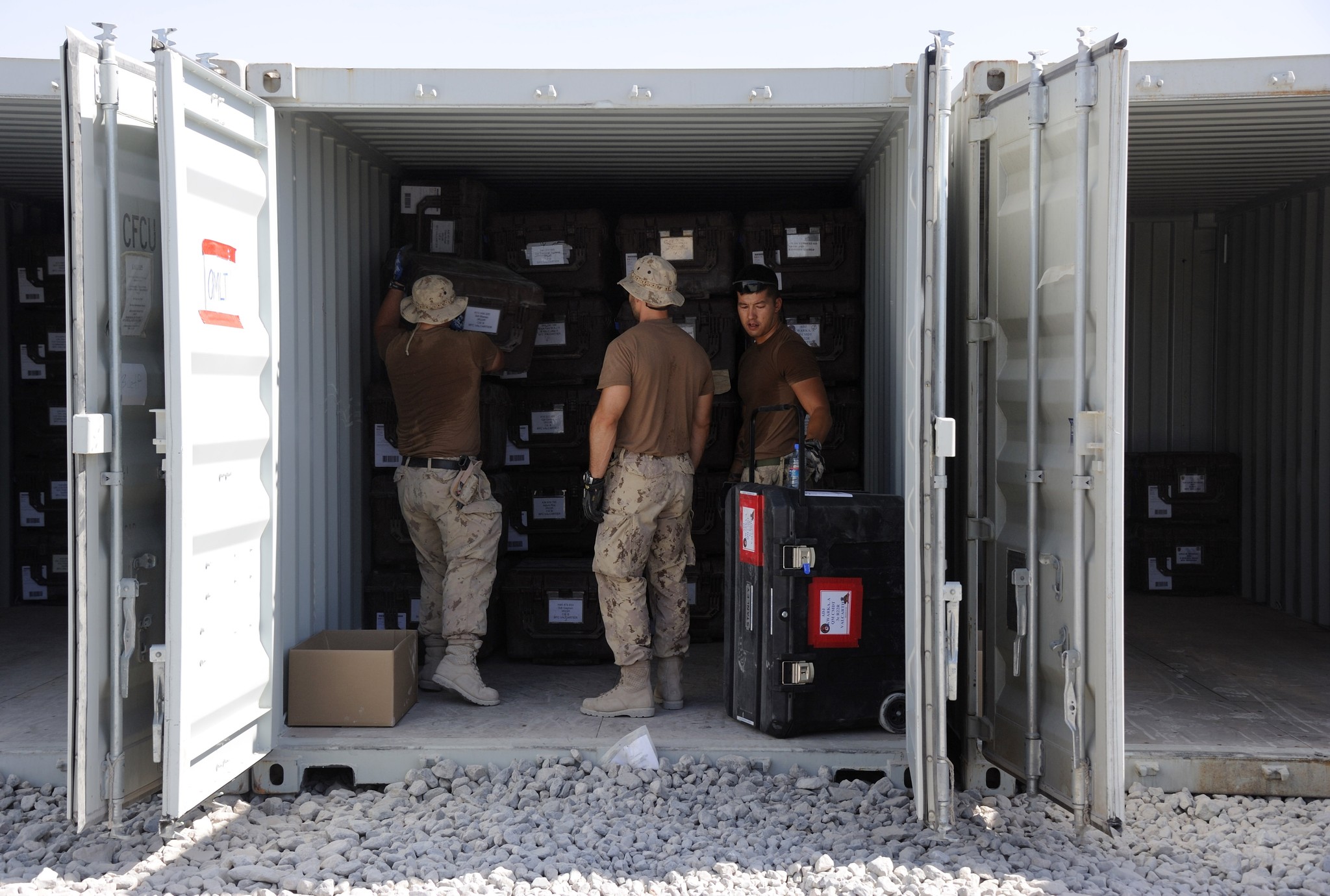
[804,438,823,452]
[583,469,605,486]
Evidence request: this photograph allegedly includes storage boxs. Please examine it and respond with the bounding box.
[1125,525,1240,598]
[1123,451,1242,529]
[362,179,908,734]
[287,629,417,727]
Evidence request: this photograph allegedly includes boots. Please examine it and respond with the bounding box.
[653,656,684,710]
[419,638,449,692]
[580,660,656,718]
[430,638,501,707]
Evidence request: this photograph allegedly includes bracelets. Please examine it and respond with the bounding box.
[388,280,406,292]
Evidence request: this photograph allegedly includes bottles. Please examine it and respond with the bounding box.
[787,444,800,489]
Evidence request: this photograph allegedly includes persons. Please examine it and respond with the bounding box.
[719,264,833,489]
[578,253,717,722]
[370,243,507,708]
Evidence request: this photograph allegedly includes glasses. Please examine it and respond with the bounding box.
[731,279,778,294]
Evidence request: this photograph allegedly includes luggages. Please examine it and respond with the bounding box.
[1122,449,1243,597]
[0,193,71,610]
[363,168,863,665]
[723,402,905,737]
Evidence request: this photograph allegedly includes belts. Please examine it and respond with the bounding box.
[744,457,791,466]
[400,456,461,471]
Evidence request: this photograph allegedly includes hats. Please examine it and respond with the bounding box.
[616,254,685,306]
[400,275,469,325]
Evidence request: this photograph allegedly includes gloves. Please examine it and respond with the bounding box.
[392,242,415,285]
[450,314,466,331]
[783,444,824,483]
[582,479,605,523]
